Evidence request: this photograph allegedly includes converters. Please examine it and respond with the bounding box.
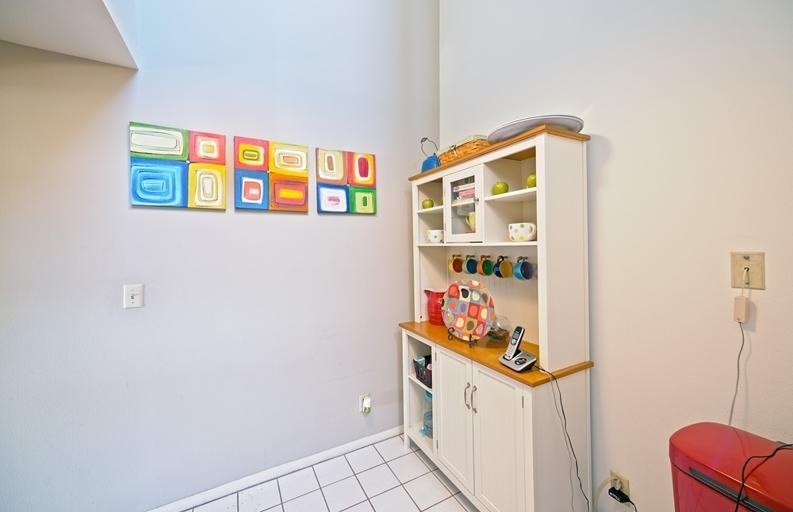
[608,487,629,503]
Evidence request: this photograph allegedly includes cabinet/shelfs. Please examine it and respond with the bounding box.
[437,345,595,512]
[408,122,590,373]
[399,323,437,464]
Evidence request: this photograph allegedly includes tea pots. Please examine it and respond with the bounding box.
[423,289,445,326]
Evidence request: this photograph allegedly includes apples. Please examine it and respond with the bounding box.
[526,174,536,188]
[492,181,508,195]
[422,198,434,208]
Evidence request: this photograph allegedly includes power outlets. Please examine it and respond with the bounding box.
[358,393,371,412]
[609,469,629,504]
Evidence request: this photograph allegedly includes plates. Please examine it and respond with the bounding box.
[485,114,584,145]
[441,279,496,341]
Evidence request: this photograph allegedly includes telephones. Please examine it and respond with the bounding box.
[498,326,537,373]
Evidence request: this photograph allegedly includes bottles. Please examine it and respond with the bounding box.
[421,153,438,172]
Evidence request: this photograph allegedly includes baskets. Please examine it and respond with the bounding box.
[414,355,432,388]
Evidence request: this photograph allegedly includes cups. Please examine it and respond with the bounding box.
[448,254,462,273]
[464,211,475,232]
[511,256,534,280]
[507,222,536,242]
[476,255,492,276]
[460,255,476,274]
[427,230,443,243]
[492,255,511,278]
